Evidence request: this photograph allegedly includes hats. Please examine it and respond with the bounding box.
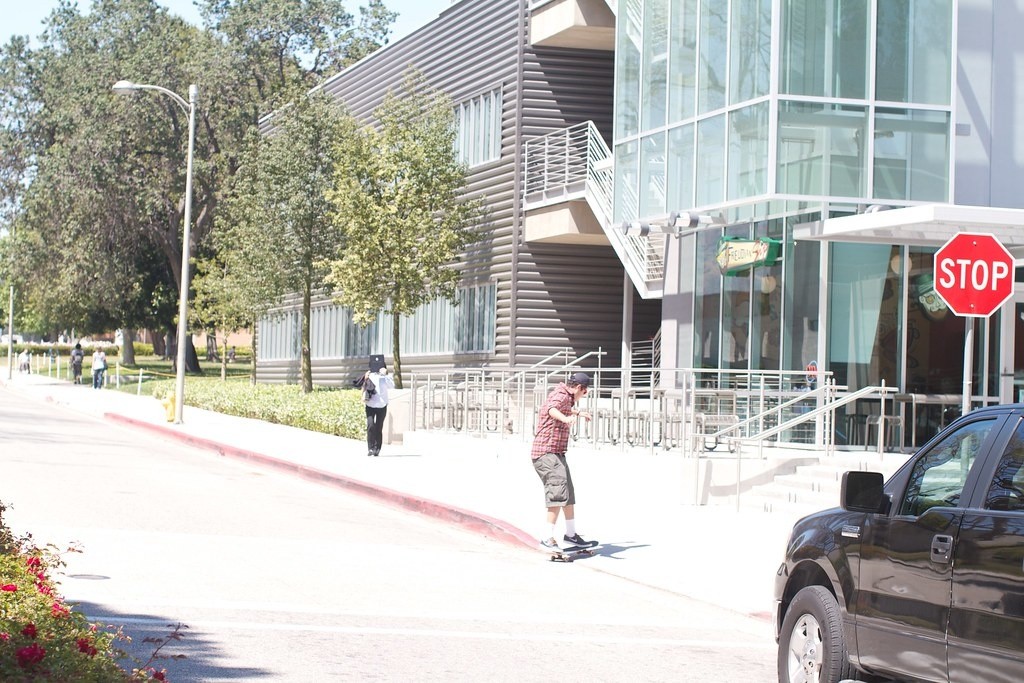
[572,373,590,386]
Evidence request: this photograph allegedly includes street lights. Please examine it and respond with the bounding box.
[110,78,200,426]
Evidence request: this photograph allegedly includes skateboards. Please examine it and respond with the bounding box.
[548,541,599,563]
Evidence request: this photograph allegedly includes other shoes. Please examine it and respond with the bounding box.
[368,448,378,455]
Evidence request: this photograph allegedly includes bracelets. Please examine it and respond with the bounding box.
[577,411,580,415]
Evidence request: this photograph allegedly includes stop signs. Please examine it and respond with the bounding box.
[933,231,1016,317]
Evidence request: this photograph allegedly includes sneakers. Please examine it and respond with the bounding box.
[563,533,592,547]
[540,538,563,552]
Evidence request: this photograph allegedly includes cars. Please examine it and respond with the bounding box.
[773,402,1024,683]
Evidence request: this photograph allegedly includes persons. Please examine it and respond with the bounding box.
[18,349,30,375]
[91,346,106,389]
[69,344,84,384]
[361,362,394,457]
[531,373,599,553]
[227,346,236,363]
